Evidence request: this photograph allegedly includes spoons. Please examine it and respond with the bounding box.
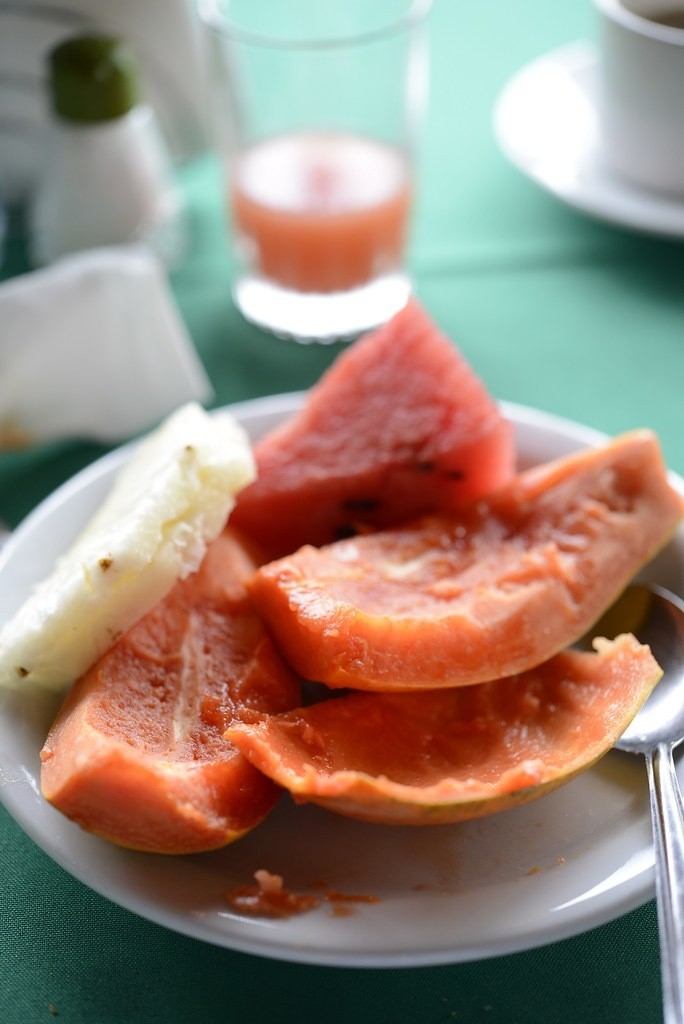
[570,573,682,1024]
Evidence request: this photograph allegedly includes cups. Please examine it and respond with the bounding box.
[193,0,425,342]
[591,0,682,195]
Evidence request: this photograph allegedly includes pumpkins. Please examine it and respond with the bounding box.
[0,299,684,856]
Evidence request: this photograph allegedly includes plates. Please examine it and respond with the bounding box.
[0,390,683,969]
[490,41,682,237]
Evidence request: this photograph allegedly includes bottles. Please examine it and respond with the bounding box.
[23,36,182,276]
